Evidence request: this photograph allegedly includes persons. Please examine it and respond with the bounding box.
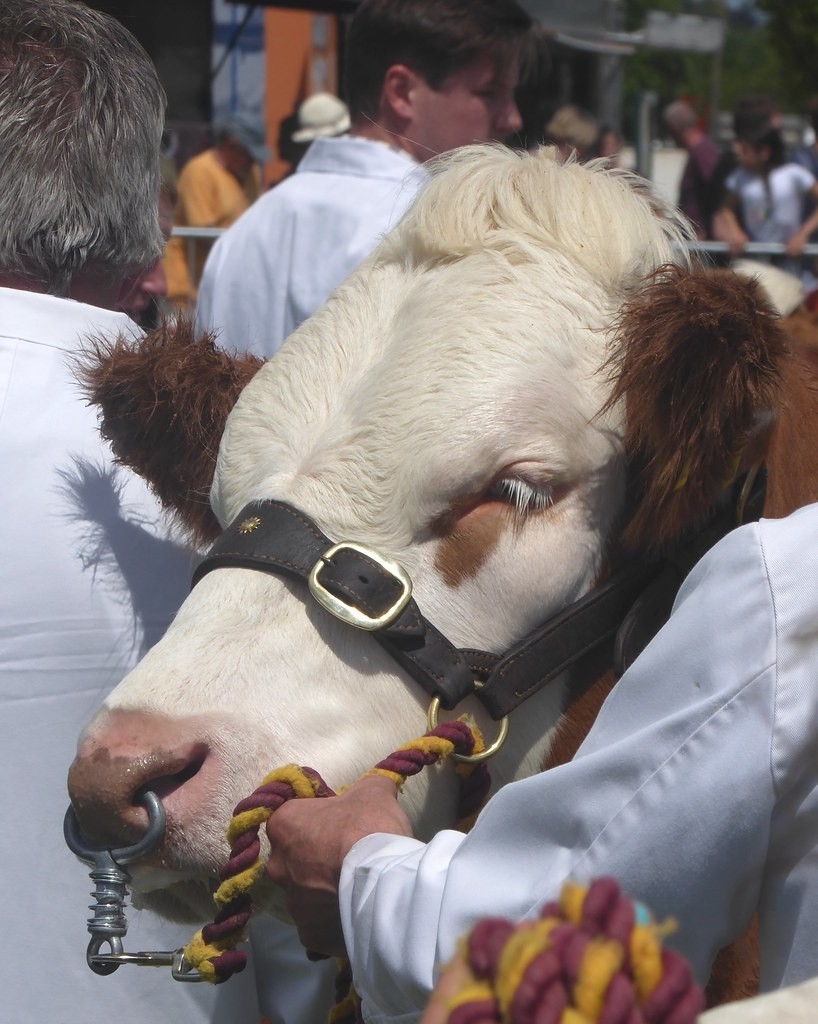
[265,498,818,995]
[544,95,818,279]
[269,91,352,187]
[0,0,337,1024]
[173,112,271,290]
[195,0,545,361]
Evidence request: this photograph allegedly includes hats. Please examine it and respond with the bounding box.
[548,109,581,137]
[291,93,352,144]
[229,114,272,162]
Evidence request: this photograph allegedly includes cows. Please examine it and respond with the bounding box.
[60,142,818,925]
